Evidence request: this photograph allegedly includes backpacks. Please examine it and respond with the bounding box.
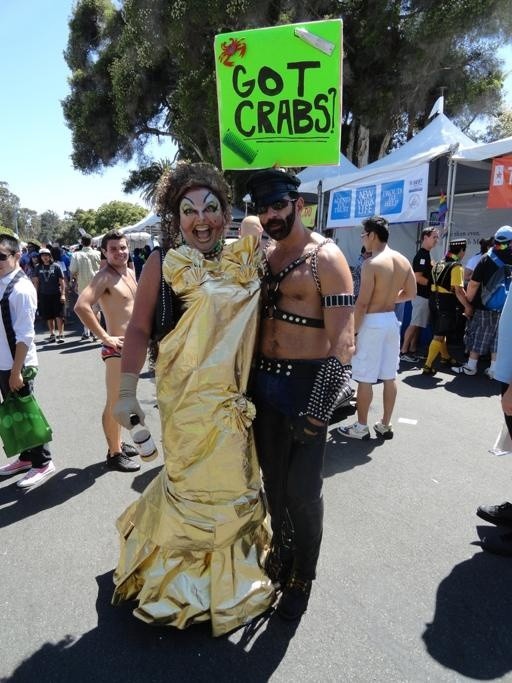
[480,250,511,312]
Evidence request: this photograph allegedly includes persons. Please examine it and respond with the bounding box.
[251,168,358,621]
[356,225,511,374]
[0,232,57,486]
[493,280,512,437]
[20,228,154,342]
[338,219,416,440]
[114,156,277,638]
[72,229,140,472]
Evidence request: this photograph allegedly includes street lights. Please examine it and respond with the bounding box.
[16,210,21,235]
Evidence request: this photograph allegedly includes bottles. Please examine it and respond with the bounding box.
[129,415,159,462]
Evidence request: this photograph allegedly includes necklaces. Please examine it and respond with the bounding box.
[182,240,222,259]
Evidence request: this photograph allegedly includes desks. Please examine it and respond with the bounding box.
[395,301,432,352]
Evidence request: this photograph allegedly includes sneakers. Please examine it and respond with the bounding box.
[0,460,32,475]
[476,502,511,557]
[374,419,393,439]
[107,444,141,471]
[337,422,370,440]
[17,462,55,487]
[50,334,56,342]
[58,336,65,342]
[278,576,312,619]
[266,543,291,579]
[398,351,477,376]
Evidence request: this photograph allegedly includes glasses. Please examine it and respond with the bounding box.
[0,253,13,261]
[256,198,298,214]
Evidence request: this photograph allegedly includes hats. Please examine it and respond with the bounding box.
[448,238,466,248]
[246,170,301,205]
[495,225,512,243]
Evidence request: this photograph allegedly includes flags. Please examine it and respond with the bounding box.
[438,188,448,223]
